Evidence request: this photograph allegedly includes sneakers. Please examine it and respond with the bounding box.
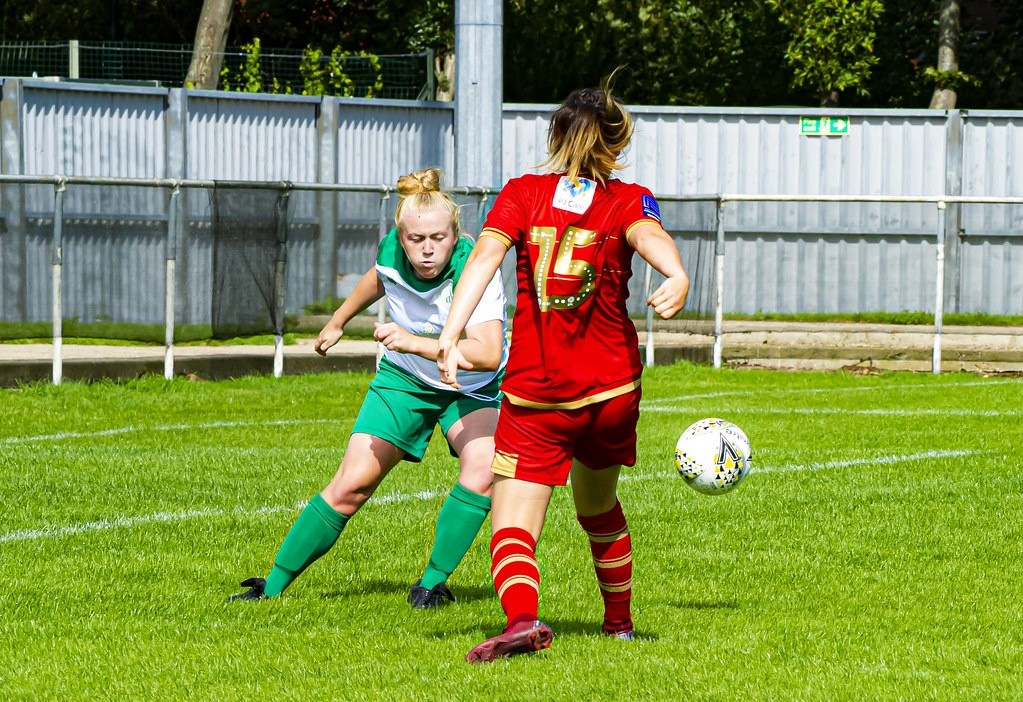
[601,615,634,641]
[223,577,269,604]
[407,579,455,611]
[464,619,553,664]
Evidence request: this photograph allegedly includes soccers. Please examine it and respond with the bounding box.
[674,417,752,496]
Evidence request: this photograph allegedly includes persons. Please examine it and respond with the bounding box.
[230,169,508,610]
[438,87,690,662]
